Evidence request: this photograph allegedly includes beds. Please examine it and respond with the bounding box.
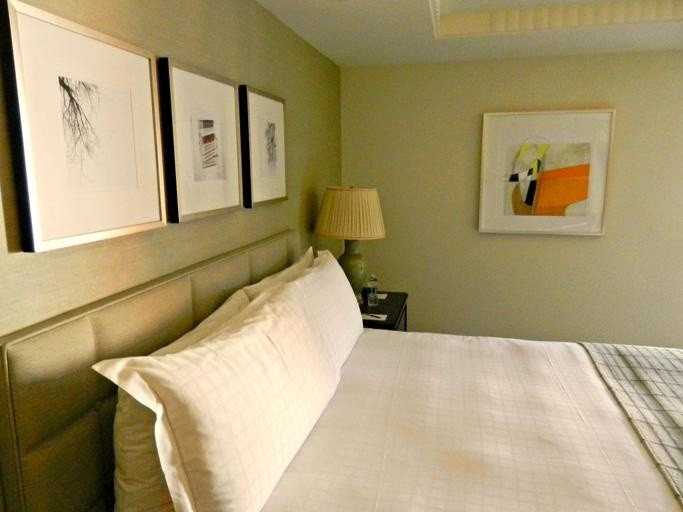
[1,228,682,512]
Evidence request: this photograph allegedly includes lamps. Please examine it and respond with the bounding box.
[312,185,386,302]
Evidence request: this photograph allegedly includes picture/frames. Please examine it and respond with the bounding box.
[161,53,243,225]
[4,0,166,254]
[478,108,615,239]
[240,84,290,209]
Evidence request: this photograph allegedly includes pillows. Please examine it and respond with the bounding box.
[108,283,341,512]
[114,290,249,512]
[243,246,314,304]
[287,247,365,370]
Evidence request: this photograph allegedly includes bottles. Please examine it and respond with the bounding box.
[367,274,378,308]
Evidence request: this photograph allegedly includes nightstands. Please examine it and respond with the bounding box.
[339,286,412,329]
